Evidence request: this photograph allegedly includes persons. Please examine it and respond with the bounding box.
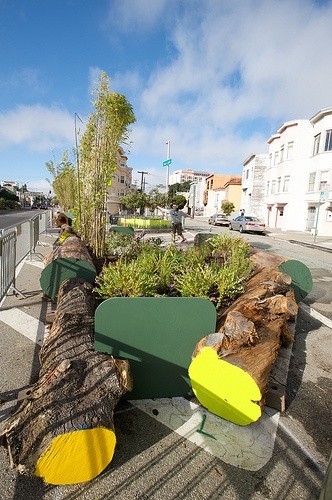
[154,202,194,243]
[241,212,244,216]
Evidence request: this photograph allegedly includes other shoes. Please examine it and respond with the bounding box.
[183,238,186,242]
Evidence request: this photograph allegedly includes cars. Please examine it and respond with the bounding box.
[229,216,266,234]
[40,205,48,210]
[31,205,38,209]
[208,214,230,227]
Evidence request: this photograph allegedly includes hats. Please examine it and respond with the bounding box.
[170,201,178,209]
